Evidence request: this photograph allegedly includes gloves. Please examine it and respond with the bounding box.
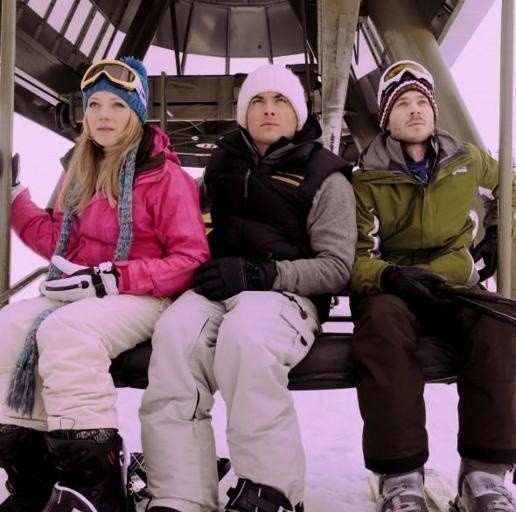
[469,227,497,280]
[381,266,449,312]
[39,254,119,302]
[9,153,25,205]
[192,256,275,300]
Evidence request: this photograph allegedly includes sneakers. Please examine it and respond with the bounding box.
[378,470,429,512]
[448,458,516,512]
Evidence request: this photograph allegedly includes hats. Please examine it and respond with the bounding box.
[236,65,310,129]
[83,57,150,124]
[379,76,438,130]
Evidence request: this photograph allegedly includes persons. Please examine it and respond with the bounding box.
[350,60,515,511]
[139,63,360,512]
[0,57,212,511]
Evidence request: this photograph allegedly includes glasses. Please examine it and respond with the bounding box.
[80,60,147,108]
[377,60,434,107]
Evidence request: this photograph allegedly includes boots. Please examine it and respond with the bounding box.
[41,427,128,512]
[1,422,55,512]
